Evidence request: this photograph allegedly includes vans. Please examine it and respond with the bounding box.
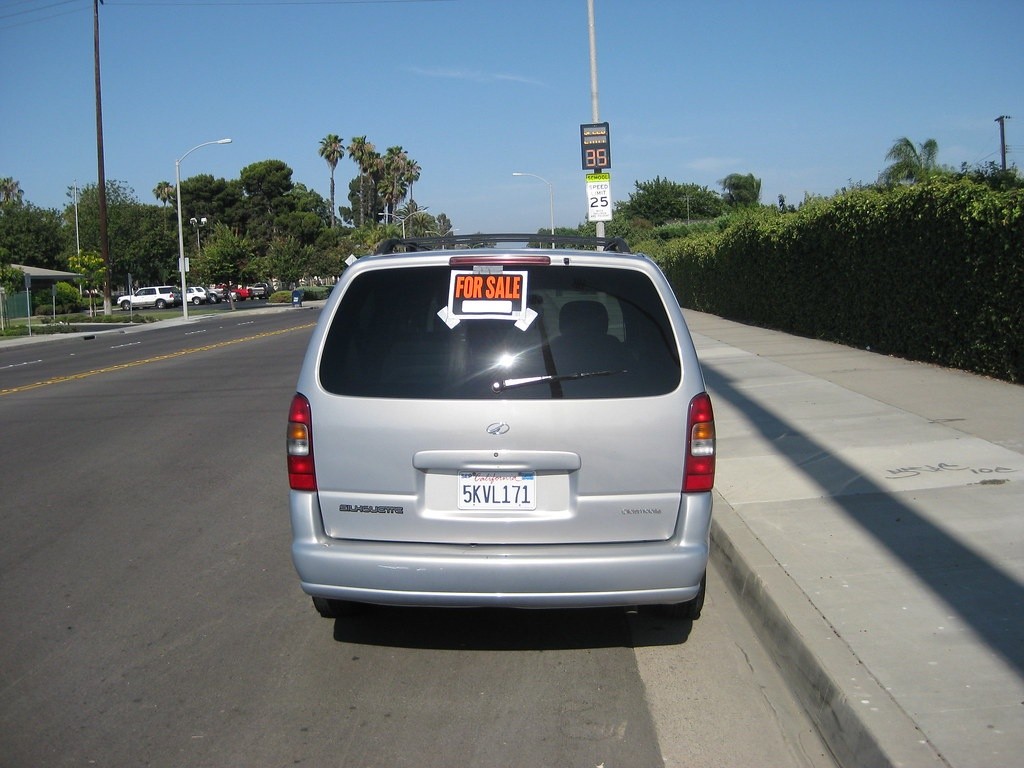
[117,286,180,310]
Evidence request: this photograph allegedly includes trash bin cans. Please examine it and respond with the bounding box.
[291,290,303,307]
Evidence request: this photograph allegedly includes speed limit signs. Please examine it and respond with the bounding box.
[587,172,613,223]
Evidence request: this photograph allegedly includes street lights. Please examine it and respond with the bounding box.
[513,172,557,249]
[425,228,459,249]
[378,210,428,252]
[190,217,207,283]
[175,138,233,320]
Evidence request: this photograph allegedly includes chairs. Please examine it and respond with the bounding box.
[550,301,622,350]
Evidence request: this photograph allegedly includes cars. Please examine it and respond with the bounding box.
[285,233,719,621]
[179,287,210,305]
[208,287,224,304]
[228,287,249,301]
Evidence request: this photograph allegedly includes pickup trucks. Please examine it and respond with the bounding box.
[247,283,274,299]
